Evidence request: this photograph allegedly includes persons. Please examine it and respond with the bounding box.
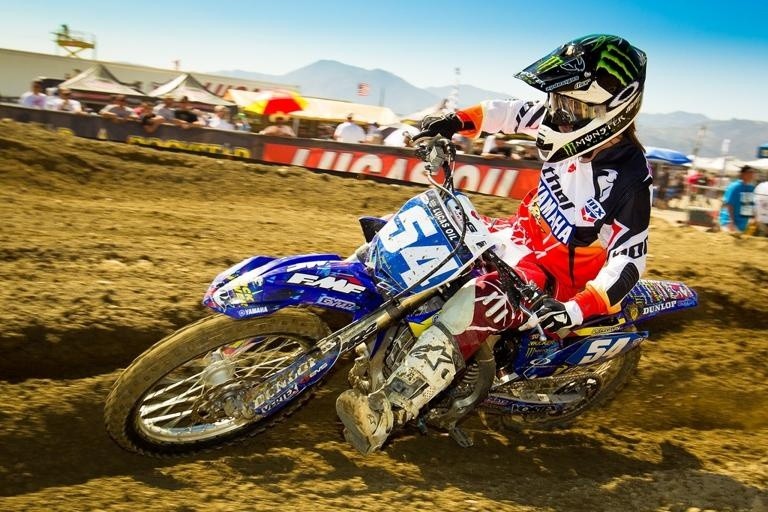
[450,129,540,161]
[98,91,251,133]
[647,160,767,237]
[334,32,656,459]
[259,111,296,136]
[20,80,82,114]
[333,114,384,144]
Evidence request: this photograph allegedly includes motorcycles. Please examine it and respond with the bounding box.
[103,114,699,462]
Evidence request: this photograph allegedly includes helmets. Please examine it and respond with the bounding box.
[511,33,647,168]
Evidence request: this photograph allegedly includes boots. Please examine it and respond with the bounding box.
[335,323,469,458]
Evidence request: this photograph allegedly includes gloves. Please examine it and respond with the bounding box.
[518,297,585,334]
[409,110,467,144]
